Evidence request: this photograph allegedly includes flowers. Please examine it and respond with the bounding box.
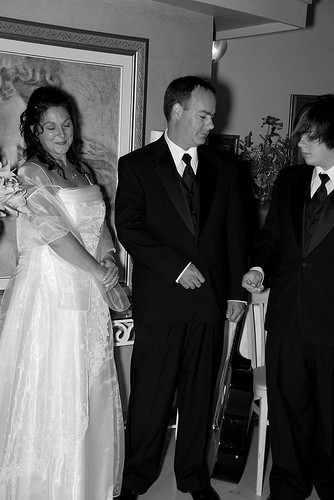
[0,160,36,219]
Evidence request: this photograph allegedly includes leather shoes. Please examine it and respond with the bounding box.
[176,483,221,500]
[113,489,139,500]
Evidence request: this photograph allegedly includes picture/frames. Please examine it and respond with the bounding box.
[0,17,150,296]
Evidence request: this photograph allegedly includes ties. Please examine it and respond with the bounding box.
[305,173,330,226]
[181,154,196,232]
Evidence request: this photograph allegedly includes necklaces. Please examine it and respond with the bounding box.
[72,169,78,180]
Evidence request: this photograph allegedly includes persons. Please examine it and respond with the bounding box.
[0,84,130,500]
[241,93,334,499]
[114,76,259,500]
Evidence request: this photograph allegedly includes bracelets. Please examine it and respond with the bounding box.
[104,253,116,263]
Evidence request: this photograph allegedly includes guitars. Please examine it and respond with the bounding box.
[207,298,253,485]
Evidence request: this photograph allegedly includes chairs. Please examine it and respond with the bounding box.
[248,289,269,496]
